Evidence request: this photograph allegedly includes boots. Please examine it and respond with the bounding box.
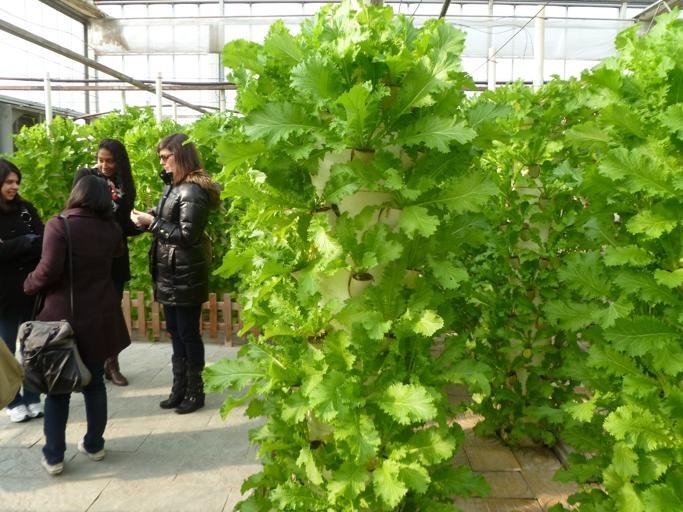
[160,356,187,408]
[174,359,205,414]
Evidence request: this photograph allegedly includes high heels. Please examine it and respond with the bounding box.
[104,356,128,386]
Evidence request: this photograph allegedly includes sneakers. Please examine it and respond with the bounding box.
[27,401,45,419]
[77,439,107,462]
[5,404,30,423]
[41,454,64,476]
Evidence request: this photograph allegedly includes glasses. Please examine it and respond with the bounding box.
[159,154,175,160]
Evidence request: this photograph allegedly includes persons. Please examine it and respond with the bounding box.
[20,173,134,475]
[128,133,223,414]
[0,156,46,423]
[71,138,143,386]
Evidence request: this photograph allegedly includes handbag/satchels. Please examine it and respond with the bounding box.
[14,320,92,397]
